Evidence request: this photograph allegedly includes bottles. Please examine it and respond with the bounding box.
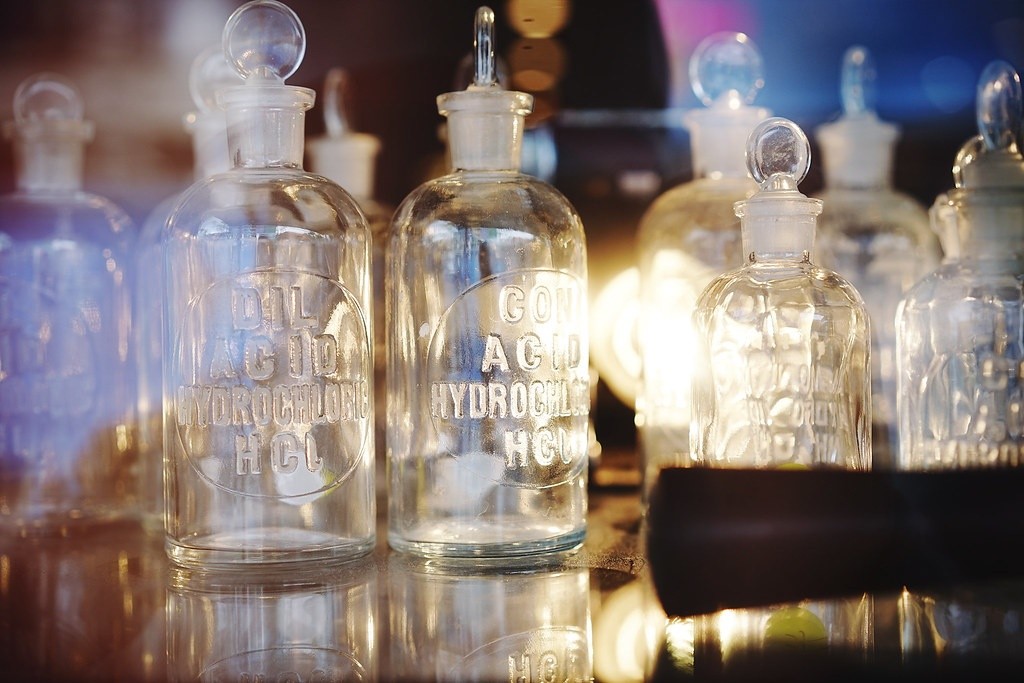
[0,1,1024,559]
